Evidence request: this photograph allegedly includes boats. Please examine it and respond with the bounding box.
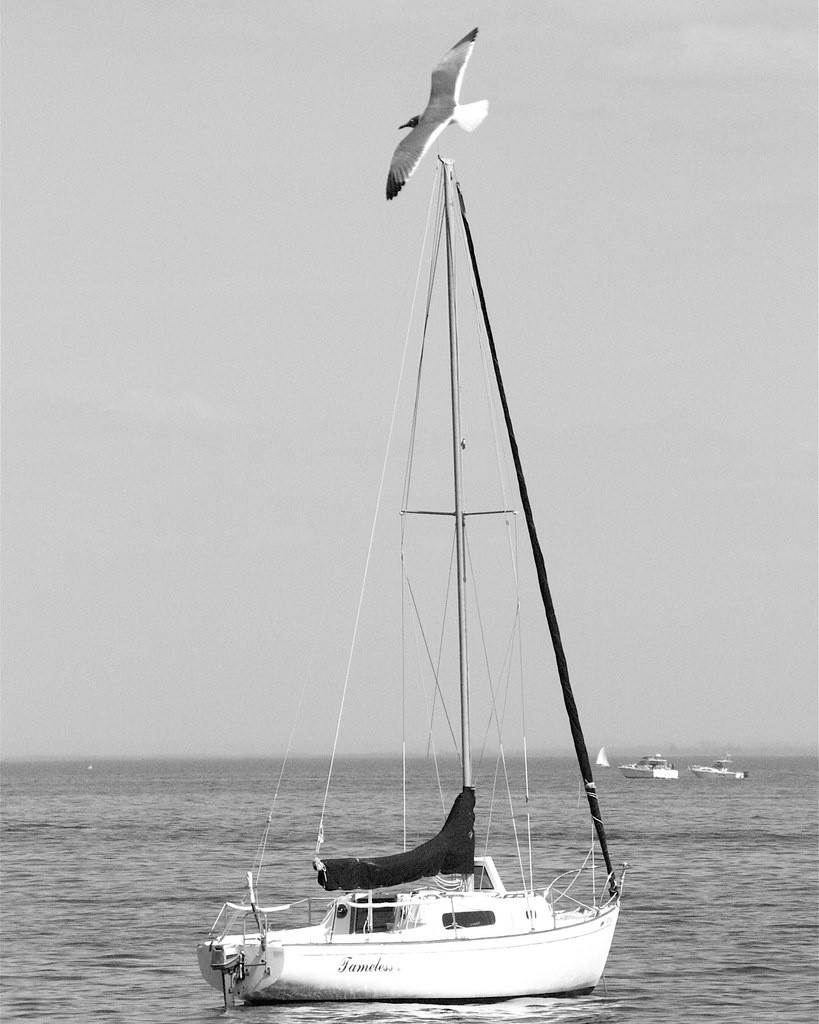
[617,753,680,780]
[687,753,749,779]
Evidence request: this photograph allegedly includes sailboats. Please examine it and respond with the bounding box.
[193,152,627,1001]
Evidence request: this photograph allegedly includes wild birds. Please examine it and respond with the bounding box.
[386,27,490,202]
